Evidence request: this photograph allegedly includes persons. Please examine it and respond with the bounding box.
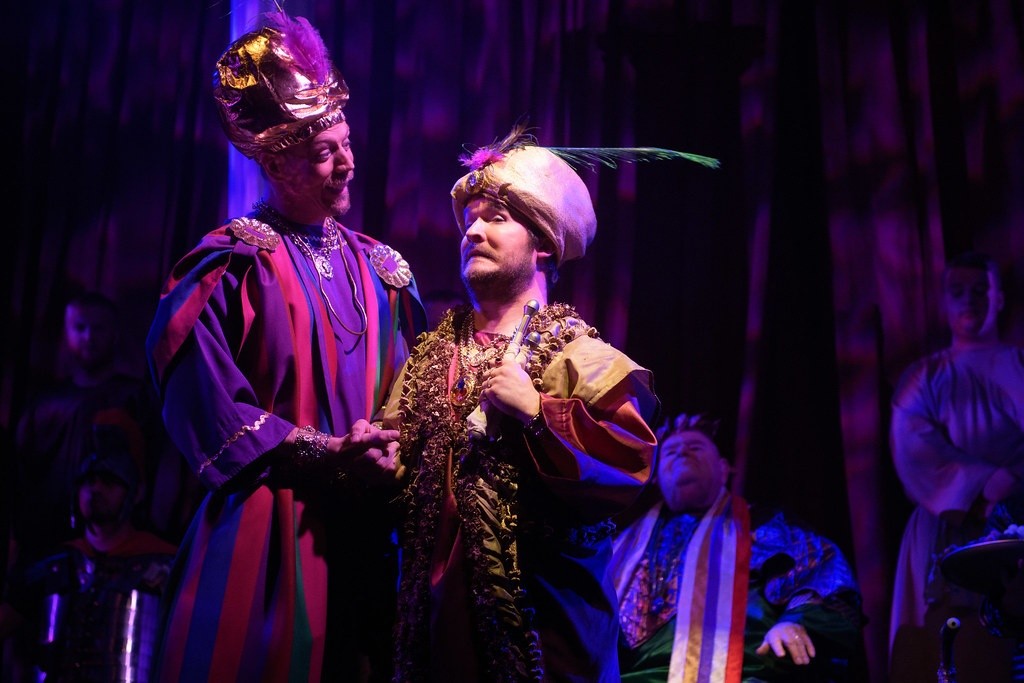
[144,42,438,681]
[604,406,857,683]
[9,284,141,452]
[883,251,1023,658]
[348,130,665,683]
[23,451,181,586]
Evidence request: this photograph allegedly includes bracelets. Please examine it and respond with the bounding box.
[522,409,543,430]
[294,425,332,460]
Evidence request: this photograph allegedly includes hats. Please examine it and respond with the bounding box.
[449,147,596,268]
[214,25,349,160]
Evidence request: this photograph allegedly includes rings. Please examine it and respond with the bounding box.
[486,376,492,388]
[494,356,503,368]
[489,367,495,377]
[792,633,802,641]
